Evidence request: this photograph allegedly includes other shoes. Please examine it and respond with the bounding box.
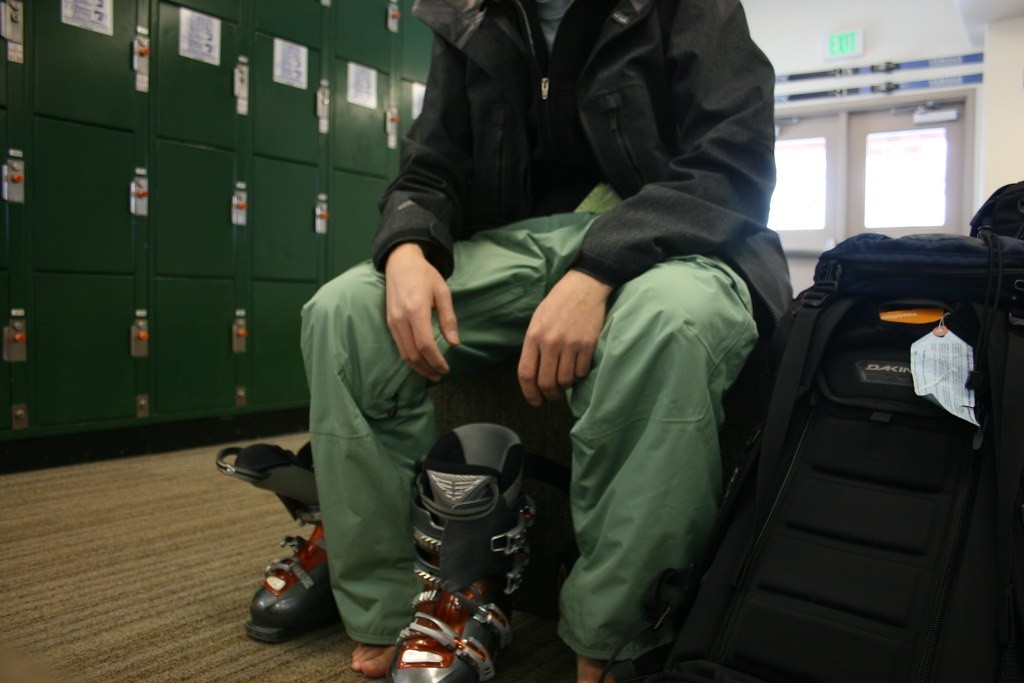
[214,438,344,639]
[389,425,530,683]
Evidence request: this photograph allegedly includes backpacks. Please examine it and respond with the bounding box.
[645,227,1023,682]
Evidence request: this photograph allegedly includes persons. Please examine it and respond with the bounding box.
[299,2,794,683]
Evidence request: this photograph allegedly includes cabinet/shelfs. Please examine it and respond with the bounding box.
[0,0,436,454]
